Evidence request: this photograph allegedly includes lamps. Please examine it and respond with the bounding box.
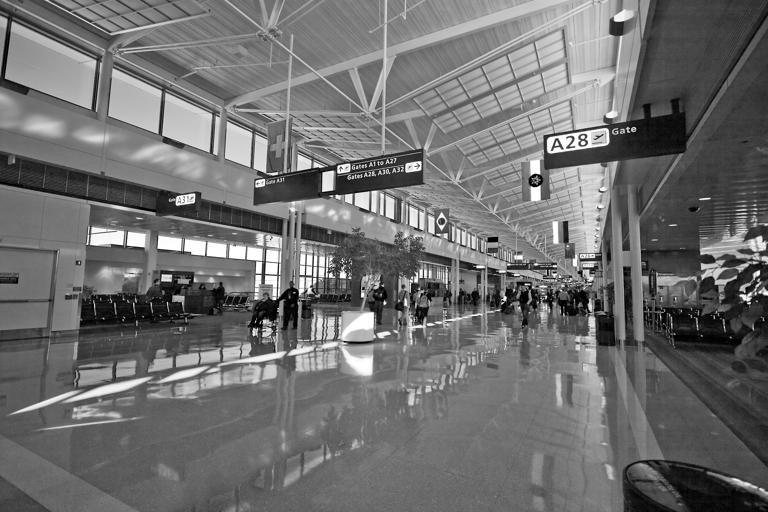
[592,10,638,239]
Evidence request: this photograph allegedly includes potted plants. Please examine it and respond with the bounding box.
[323,343,418,456]
[326,223,429,344]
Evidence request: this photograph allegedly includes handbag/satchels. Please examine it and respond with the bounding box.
[394,303,404,310]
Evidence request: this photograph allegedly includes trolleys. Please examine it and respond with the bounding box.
[248,330,276,348]
[253,307,277,329]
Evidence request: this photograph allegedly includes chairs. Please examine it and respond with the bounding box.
[307,291,350,305]
[78,287,261,361]
[644,302,764,350]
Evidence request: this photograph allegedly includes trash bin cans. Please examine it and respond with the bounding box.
[622,460,768,512]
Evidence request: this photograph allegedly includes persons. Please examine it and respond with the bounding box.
[122,277,137,294]
[368,282,592,329]
[301,285,318,299]
[173,285,184,295]
[199,283,206,290]
[146,278,162,297]
[213,281,224,314]
[246,292,277,329]
[277,281,299,330]
